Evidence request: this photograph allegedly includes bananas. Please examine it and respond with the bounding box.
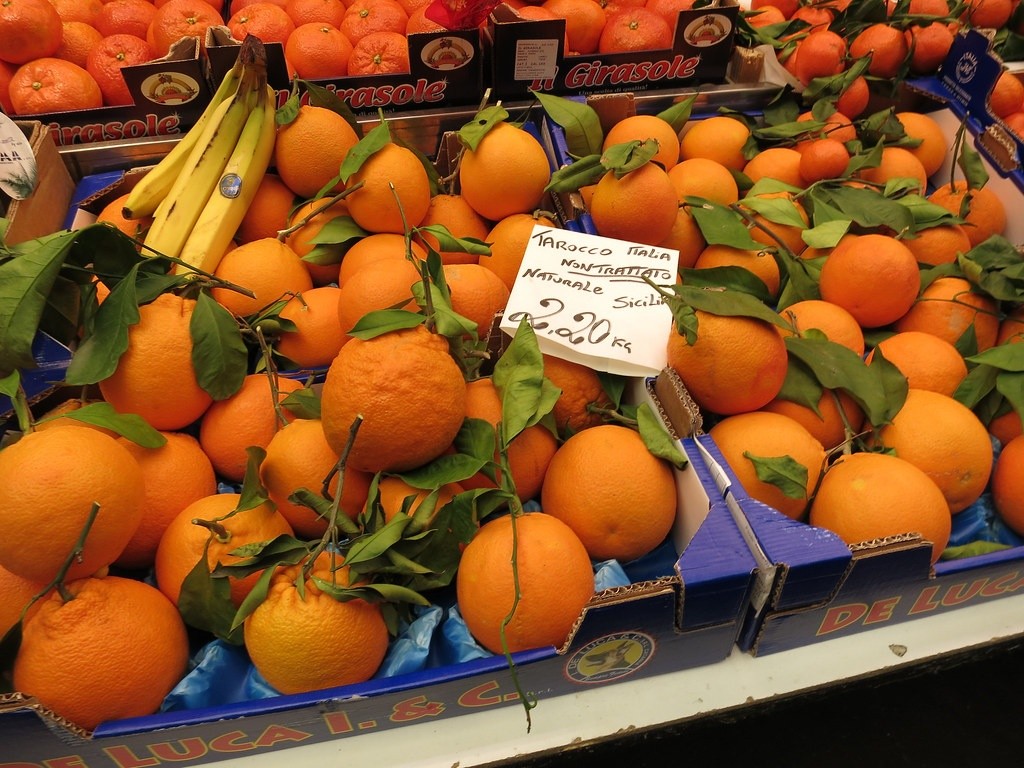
[122,34,275,282]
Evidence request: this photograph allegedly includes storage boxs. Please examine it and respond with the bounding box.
[0,0,1024,768]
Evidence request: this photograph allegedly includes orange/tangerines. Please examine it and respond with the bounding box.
[0,105,1024,731]
[0,0,1024,137]
